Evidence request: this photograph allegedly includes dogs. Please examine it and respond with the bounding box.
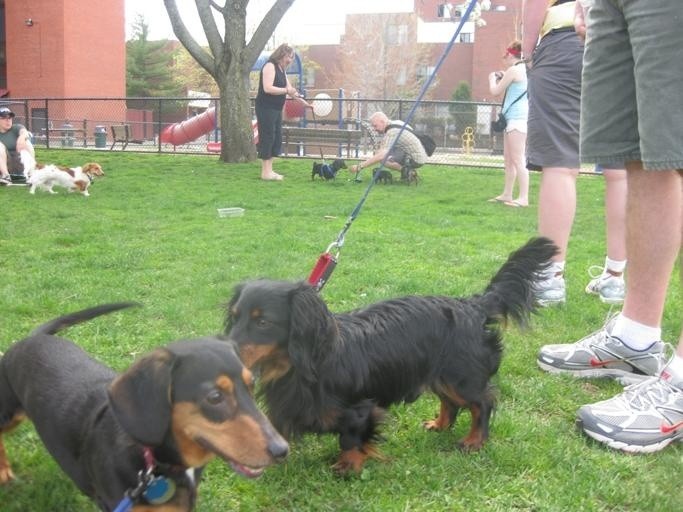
[401,167,418,187]
[0,301,289,512]
[369,168,392,185]
[312,159,347,183]
[223,239,561,481]
[20,149,104,197]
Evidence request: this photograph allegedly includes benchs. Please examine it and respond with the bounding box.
[35,126,86,147]
[110,124,144,151]
[282,127,362,158]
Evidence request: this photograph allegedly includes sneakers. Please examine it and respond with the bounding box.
[573,344,683,454]
[399,166,417,185]
[528,272,566,309]
[534,305,666,386]
[585,263,624,306]
[0,175,12,185]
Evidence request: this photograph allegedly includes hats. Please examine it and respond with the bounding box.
[0,107,14,118]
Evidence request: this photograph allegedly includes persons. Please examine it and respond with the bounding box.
[254,42,314,183]
[0,105,37,185]
[519,0,627,307]
[488,40,530,208]
[537,0,683,453]
[348,110,429,179]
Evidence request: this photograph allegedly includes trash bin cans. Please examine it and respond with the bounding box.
[94,125,108,148]
[61,119,75,148]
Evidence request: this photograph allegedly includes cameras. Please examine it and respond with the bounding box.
[495,71,504,79]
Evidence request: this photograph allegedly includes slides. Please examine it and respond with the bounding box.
[207,124,259,152]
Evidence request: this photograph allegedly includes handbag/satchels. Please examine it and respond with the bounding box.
[490,113,506,133]
[419,135,435,157]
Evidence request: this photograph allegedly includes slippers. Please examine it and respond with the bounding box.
[260,173,284,182]
[486,198,512,204]
[502,201,529,208]
